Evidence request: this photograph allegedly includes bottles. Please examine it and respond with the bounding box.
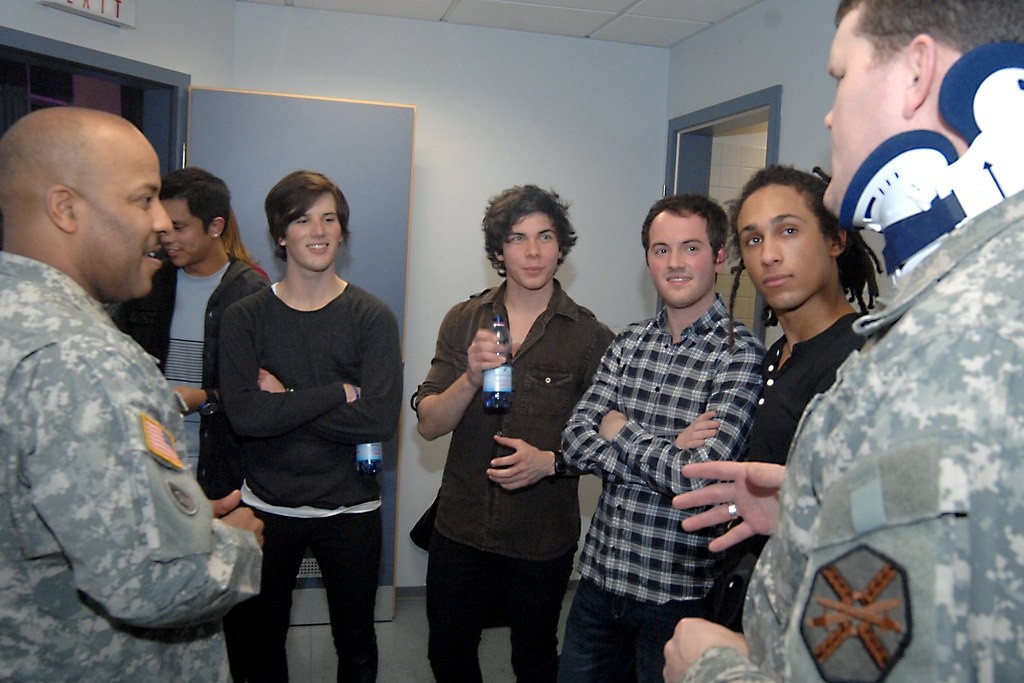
[482,314,513,410]
[355,444,384,474]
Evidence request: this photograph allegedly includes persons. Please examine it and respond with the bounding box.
[661,0,1024,683]
[220,172,403,683]
[0,106,266,683]
[698,163,881,637]
[555,195,764,683]
[407,186,616,683]
[112,168,269,499]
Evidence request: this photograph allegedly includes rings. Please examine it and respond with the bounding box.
[728,502,738,520]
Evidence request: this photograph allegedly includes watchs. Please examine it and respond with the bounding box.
[285,384,295,393]
[201,387,221,417]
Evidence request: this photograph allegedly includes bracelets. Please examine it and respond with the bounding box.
[350,384,359,401]
